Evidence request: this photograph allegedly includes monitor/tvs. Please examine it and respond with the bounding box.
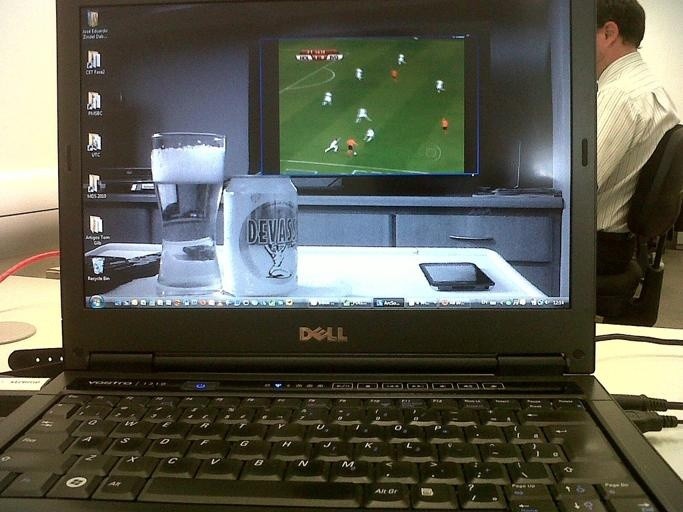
[248,22,483,196]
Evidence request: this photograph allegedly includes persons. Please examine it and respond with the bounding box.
[362,128,374,144]
[390,67,399,83]
[440,115,449,133]
[354,65,363,81]
[435,79,445,94]
[320,90,335,108]
[354,105,373,124]
[344,136,359,157]
[396,52,407,64]
[596,0,678,276]
[323,136,342,154]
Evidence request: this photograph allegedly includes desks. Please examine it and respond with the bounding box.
[0,272,683,511]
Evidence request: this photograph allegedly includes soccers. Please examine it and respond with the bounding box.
[353,151,358,155]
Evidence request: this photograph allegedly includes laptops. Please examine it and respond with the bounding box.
[1,0,683,512]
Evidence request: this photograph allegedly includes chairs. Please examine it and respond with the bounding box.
[595,124,683,329]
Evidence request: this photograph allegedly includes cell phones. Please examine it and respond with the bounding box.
[418,262,495,291]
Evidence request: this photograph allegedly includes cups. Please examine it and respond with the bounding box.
[149,130,226,299]
